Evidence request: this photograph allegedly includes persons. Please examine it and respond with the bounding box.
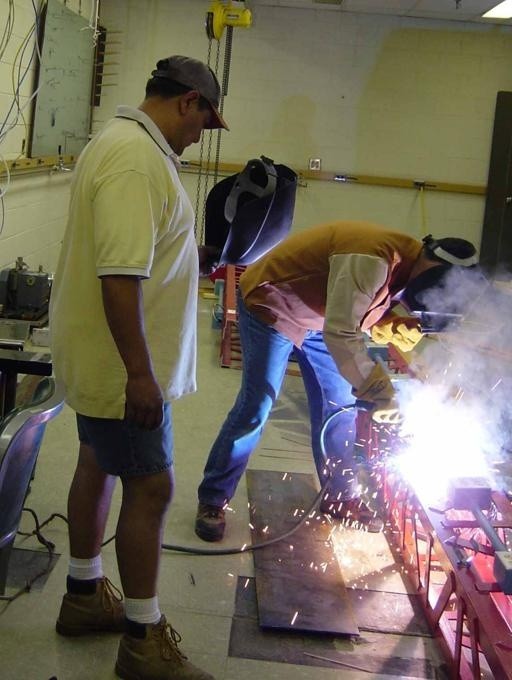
[193,219,484,546]
[46,56,227,680]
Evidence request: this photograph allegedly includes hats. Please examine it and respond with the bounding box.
[150,54,231,133]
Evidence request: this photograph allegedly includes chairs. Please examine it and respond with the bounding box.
[0,374,67,600]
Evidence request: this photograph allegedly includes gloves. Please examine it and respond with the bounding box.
[369,311,425,355]
[351,359,407,425]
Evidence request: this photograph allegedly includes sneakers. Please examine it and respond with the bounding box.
[195,499,228,544]
[115,613,216,680]
[55,575,126,639]
[318,494,385,533]
